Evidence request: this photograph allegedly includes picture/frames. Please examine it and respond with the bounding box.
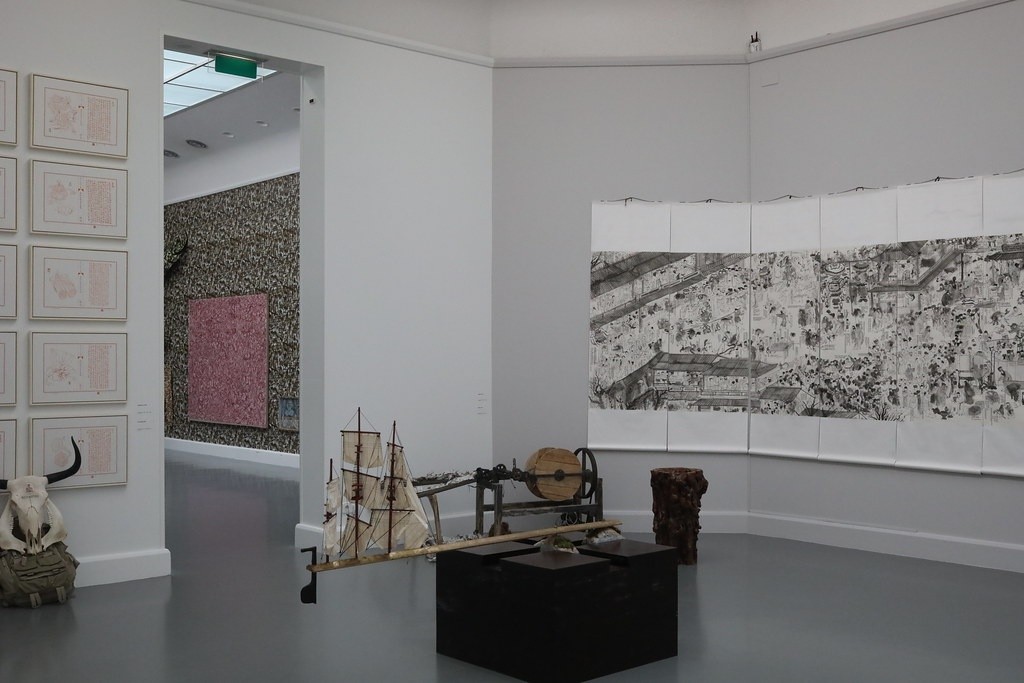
[29,158,130,241]
[28,330,129,406]
[0,66,19,497]
[28,72,130,160]
[29,413,128,492]
[28,244,130,322]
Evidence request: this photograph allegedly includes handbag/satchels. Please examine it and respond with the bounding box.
[0,541,80,608]
